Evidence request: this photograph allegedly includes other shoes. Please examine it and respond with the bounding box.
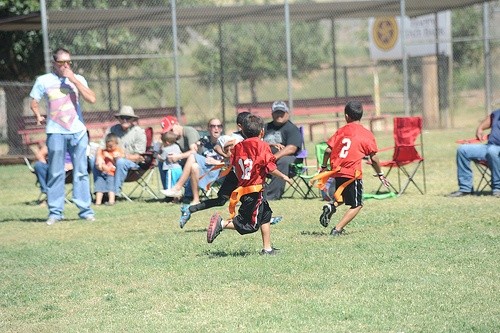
[261,247,280,256]
[319,203,336,228]
[492,189,500,199]
[207,212,223,244]
[160,187,183,198]
[330,226,349,236]
[86,214,96,220]
[443,191,473,198]
[190,201,200,206]
[46,218,63,226]
[179,204,191,229]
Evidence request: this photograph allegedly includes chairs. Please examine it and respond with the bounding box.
[27,116,491,205]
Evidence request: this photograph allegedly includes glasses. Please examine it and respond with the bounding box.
[209,124,222,130]
[54,60,73,65]
[120,115,131,121]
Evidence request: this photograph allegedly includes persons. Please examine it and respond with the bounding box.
[207,114,293,256]
[33,145,73,204]
[263,101,303,201]
[445,109,500,198]
[159,115,201,203]
[29,49,97,225]
[89,105,147,206]
[160,118,233,206]
[157,132,183,203]
[181,112,284,229]
[95,133,145,205]
[308,100,390,236]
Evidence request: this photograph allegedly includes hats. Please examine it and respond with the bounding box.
[114,105,139,121]
[272,100,288,113]
[159,115,177,134]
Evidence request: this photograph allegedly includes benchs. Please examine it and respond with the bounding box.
[235,94,390,142]
[17,105,187,155]
